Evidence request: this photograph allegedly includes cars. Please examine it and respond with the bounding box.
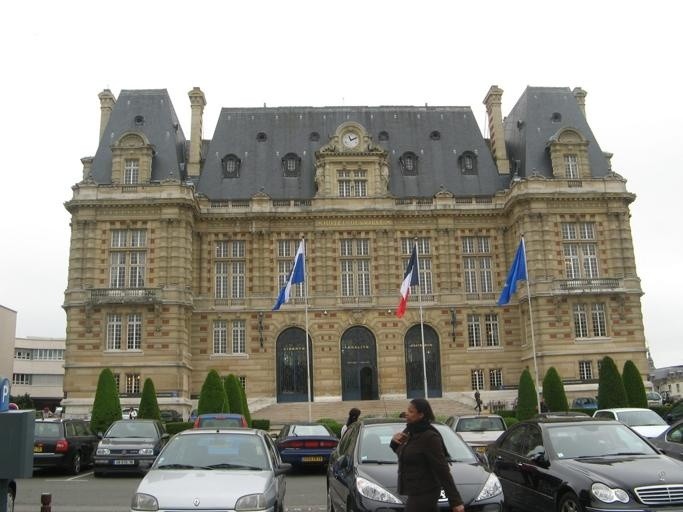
[647,391,683,406]
[572,397,598,408]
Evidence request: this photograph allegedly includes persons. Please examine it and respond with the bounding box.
[41,404,53,418]
[390,399,465,512]
[474,388,482,412]
[340,408,361,439]
[129,408,137,420]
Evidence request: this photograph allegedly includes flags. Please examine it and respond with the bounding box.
[396,245,419,318]
[271,241,305,310]
[498,239,527,304]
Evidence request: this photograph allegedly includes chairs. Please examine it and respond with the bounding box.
[180,444,258,466]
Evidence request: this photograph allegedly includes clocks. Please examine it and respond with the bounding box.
[340,130,360,149]
